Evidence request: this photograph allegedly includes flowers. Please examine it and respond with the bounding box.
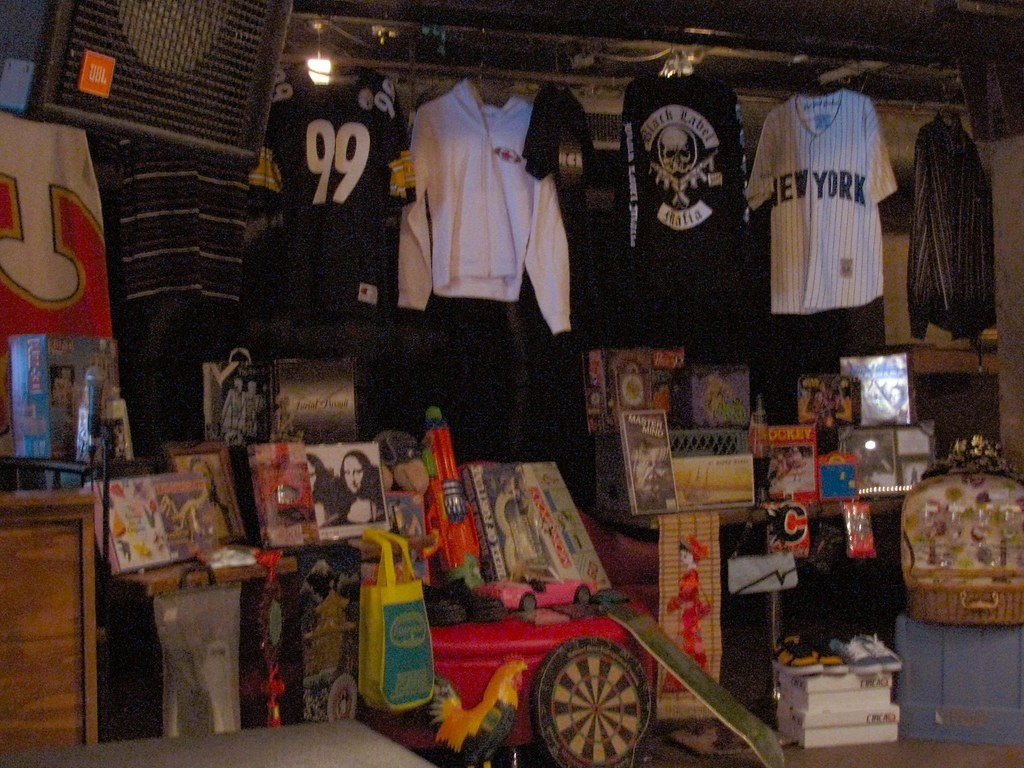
[934,433,1014,476]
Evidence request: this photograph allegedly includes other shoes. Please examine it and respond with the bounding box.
[451,576,511,626]
[408,583,467,627]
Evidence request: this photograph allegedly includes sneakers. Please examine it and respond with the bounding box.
[771,634,823,676]
[828,637,885,676]
[811,637,850,677]
[864,636,904,675]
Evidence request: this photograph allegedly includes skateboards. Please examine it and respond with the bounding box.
[606,606,785,768]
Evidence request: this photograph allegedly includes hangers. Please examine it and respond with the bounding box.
[656,42,693,79]
[470,40,511,103]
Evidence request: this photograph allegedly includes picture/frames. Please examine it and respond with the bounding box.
[838,421,938,500]
[158,439,250,543]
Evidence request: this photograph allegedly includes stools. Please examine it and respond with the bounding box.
[360,597,654,768]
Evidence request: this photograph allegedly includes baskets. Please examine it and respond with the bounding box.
[902,570,1022,629]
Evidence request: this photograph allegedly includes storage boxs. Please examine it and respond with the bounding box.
[894,612,1024,746]
[774,667,893,712]
[776,700,902,749]
[580,336,915,518]
[894,468,1022,627]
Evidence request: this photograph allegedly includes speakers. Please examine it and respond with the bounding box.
[0,0,293,163]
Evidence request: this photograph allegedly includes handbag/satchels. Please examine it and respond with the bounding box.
[358,525,439,715]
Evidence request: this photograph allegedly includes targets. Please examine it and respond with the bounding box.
[529,636,651,768]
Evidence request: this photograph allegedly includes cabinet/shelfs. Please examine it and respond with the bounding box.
[1,485,97,756]
